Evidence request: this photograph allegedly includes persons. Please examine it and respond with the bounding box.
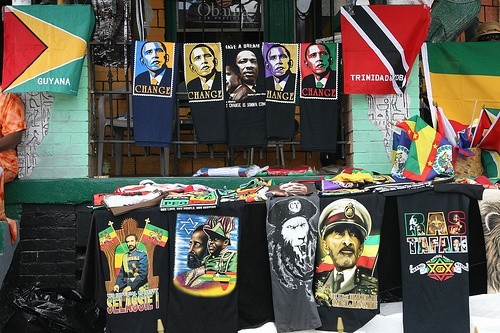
[0,81,27,245]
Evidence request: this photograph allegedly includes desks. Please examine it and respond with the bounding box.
[76,183,488,333]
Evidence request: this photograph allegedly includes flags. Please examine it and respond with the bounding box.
[339,5,431,94]
[2,4,95,98]
[391,101,500,185]
